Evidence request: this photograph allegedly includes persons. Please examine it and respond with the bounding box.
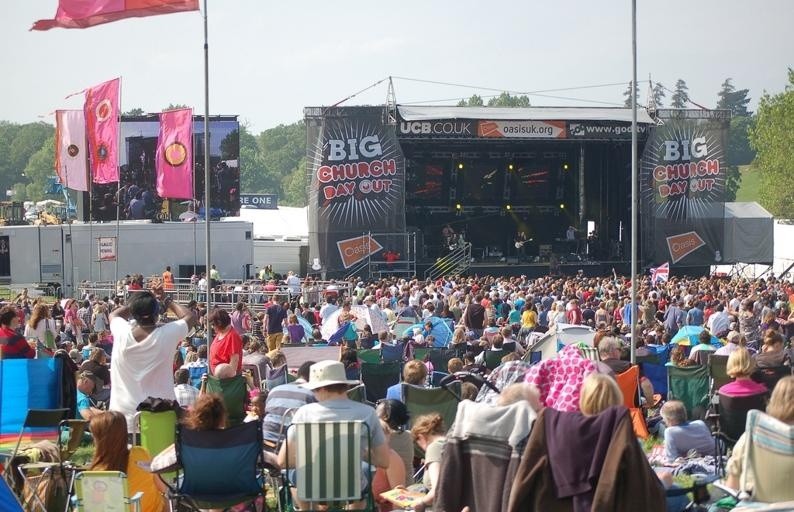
[1,265,793,510]
[96,155,232,218]
[566,225,578,240]
[442,224,465,252]
[382,249,402,270]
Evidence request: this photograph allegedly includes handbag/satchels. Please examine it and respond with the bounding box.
[44,329,55,349]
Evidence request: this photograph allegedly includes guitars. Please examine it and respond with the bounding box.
[515,239,532,249]
[449,244,458,251]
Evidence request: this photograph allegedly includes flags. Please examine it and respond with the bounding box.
[155,107,193,200]
[53,109,88,192]
[32,0,200,32]
[84,78,121,184]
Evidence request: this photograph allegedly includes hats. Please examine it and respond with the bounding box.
[68,349,82,364]
[297,360,360,391]
[363,295,373,303]
[357,281,363,287]
